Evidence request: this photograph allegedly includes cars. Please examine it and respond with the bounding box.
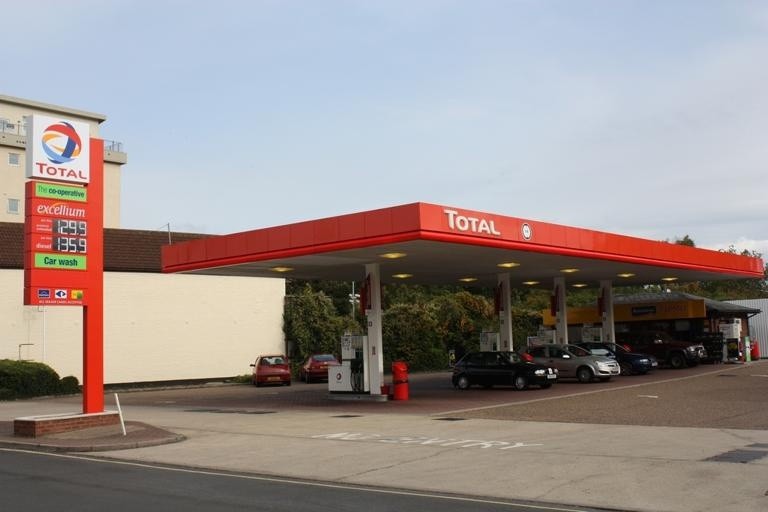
[452,331,709,391]
[249,354,292,387]
[298,352,341,384]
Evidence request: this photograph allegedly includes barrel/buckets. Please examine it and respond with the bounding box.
[381,382,390,395]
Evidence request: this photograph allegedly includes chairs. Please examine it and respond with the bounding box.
[261,359,270,365]
[274,359,282,363]
